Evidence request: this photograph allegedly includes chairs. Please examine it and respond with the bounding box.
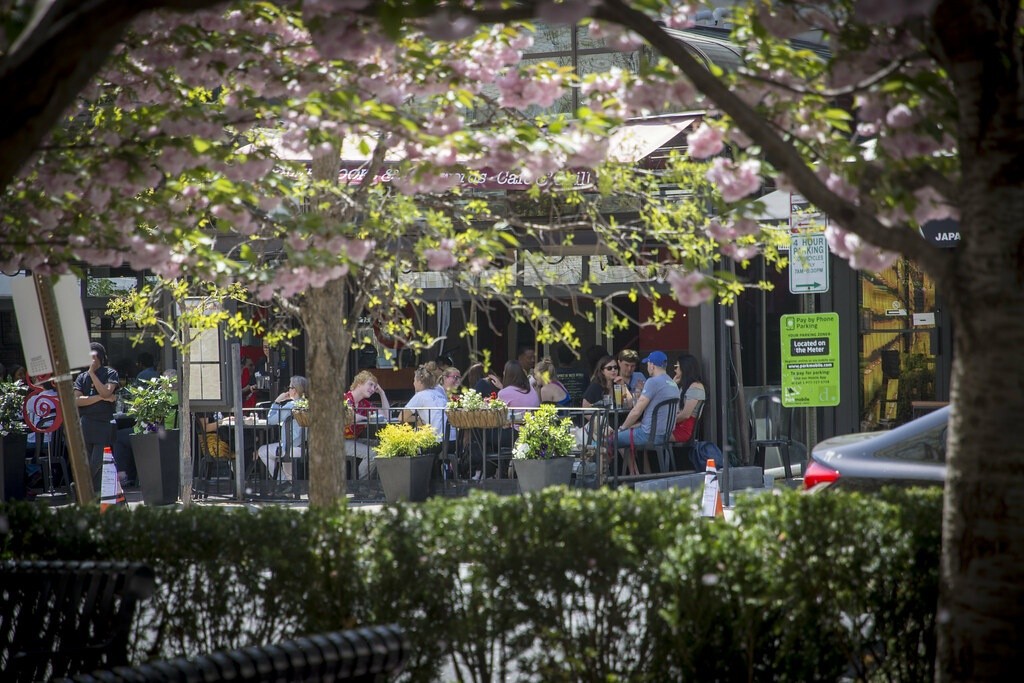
[27,378,709,498]
[0,561,149,683]
[749,389,807,478]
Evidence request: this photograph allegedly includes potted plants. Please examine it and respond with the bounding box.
[372,422,444,503]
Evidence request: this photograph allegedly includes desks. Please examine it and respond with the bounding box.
[220,423,276,494]
[355,421,402,425]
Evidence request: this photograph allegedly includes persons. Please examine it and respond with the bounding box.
[242,340,280,393]
[135,352,161,391]
[4,364,28,503]
[605,351,680,471]
[398,347,572,479]
[258,375,308,493]
[581,355,632,420]
[70,342,120,502]
[343,370,392,480]
[195,412,235,479]
[667,355,706,442]
[617,349,646,409]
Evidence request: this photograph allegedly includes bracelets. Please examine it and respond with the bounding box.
[276,402,279,404]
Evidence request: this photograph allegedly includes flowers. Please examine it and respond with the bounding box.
[124,375,178,434]
[0,374,30,437]
[445,387,502,410]
[293,399,353,415]
[513,404,576,461]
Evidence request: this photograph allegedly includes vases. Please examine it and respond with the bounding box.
[0,433,28,502]
[293,410,353,425]
[130,427,180,506]
[445,406,510,428]
[516,455,575,495]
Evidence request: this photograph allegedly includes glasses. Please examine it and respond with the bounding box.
[447,375,461,380]
[287,386,293,389]
[674,365,680,369]
[603,366,618,370]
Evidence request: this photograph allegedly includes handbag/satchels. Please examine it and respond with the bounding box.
[690,441,723,472]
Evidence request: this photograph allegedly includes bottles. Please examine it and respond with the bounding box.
[116,392,124,413]
[368,410,376,421]
[623,384,629,410]
[250,413,259,424]
[89,384,96,396]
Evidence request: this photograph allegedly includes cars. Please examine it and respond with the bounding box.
[802,405,950,497]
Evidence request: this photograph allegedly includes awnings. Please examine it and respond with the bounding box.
[234,115,699,190]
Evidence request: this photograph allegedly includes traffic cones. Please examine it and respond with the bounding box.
[99,447,127,511]
[704,459,726,521]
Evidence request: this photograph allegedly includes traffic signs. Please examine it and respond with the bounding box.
[789,234,830,294]
[789,191,828,233]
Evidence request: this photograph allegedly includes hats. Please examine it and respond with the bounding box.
[641,351,667,366]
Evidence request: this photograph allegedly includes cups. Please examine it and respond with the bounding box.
[603,384,622,409]
[762,474,774,490]
[632,392,639,408]
[256,376,270,389]
